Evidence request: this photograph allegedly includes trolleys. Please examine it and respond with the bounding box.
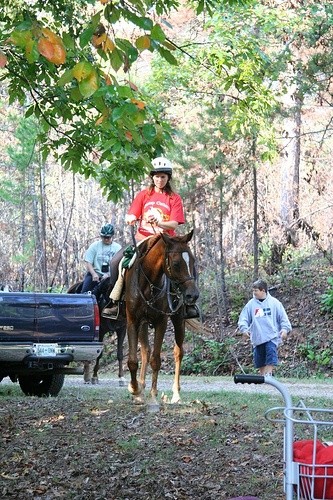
[235,374,333,500]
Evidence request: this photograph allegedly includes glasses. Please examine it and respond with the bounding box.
[102,236,109,239]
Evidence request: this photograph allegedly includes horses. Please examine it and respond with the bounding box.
[66,229,200,406]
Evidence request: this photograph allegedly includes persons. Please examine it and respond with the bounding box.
[80,223,122,295]
[237,279,292,376]
[102,156,199,317]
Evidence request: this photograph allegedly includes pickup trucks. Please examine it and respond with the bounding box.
[0,291,105,398]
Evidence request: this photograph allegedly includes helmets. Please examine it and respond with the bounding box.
[148,157,172,176]
[100,224,114,237]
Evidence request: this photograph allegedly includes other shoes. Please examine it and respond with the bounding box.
[102,301,118,315]
[186,305,197,318]
